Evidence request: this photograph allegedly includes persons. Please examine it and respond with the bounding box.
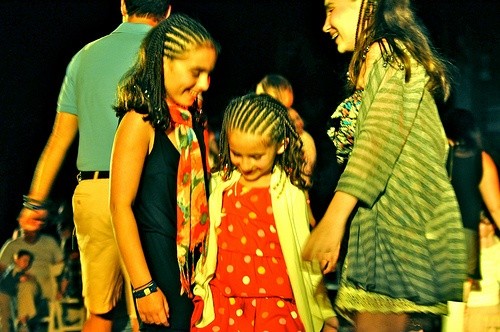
[17,0,172,331]
[0,208,87,332]
[300,0,468,332]
[441,104,500,332]
[109,15,222,332]
[190,93,341,332]
[255,73,316,229]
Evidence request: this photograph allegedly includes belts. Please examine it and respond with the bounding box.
[81,171,109,179]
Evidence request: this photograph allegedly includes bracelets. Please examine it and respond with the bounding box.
[132,279,158,299]
[22,194,49,210]
